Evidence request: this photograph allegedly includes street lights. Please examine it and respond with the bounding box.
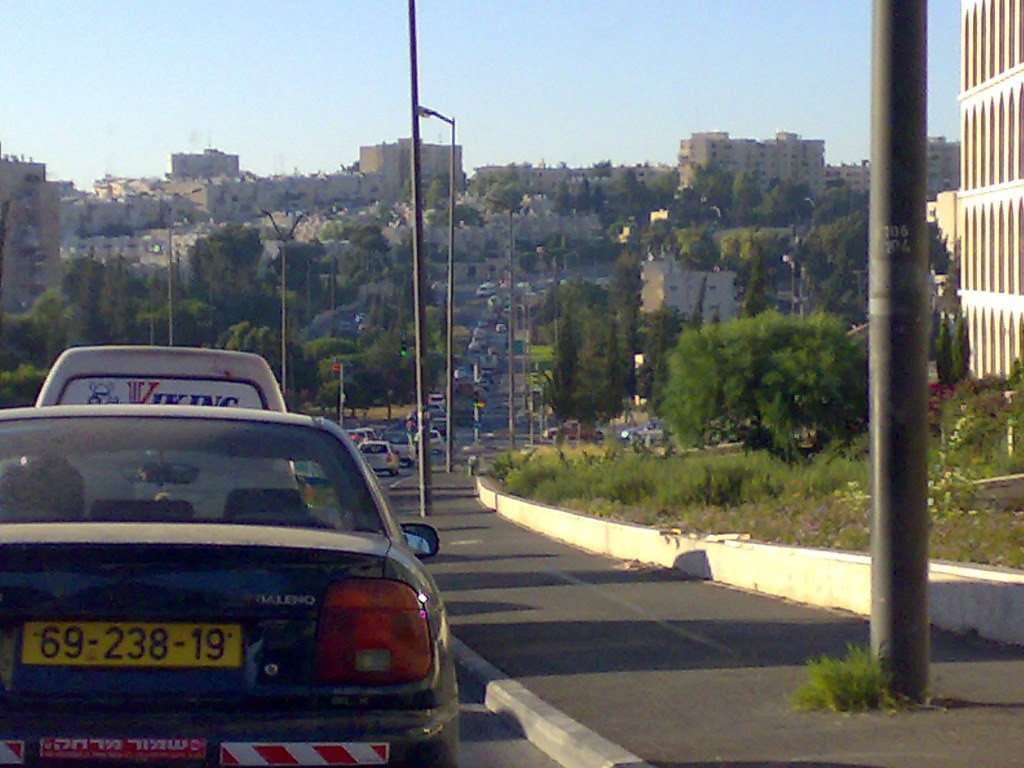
[138,187,204,348]
[415,103,456,472]
[259,207,312,394]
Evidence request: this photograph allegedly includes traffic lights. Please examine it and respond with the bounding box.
[401,344,408,357]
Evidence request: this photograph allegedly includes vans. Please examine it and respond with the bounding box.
[29,345,295,524]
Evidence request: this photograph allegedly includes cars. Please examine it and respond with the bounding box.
[379,430,416,467]
[0,405,463,768]
[544,421,595,441]
[343,427,376,449]
[355,440,400,476]
[620,422,666,442]
[413,429,444,453]
[426,279,521,437]
